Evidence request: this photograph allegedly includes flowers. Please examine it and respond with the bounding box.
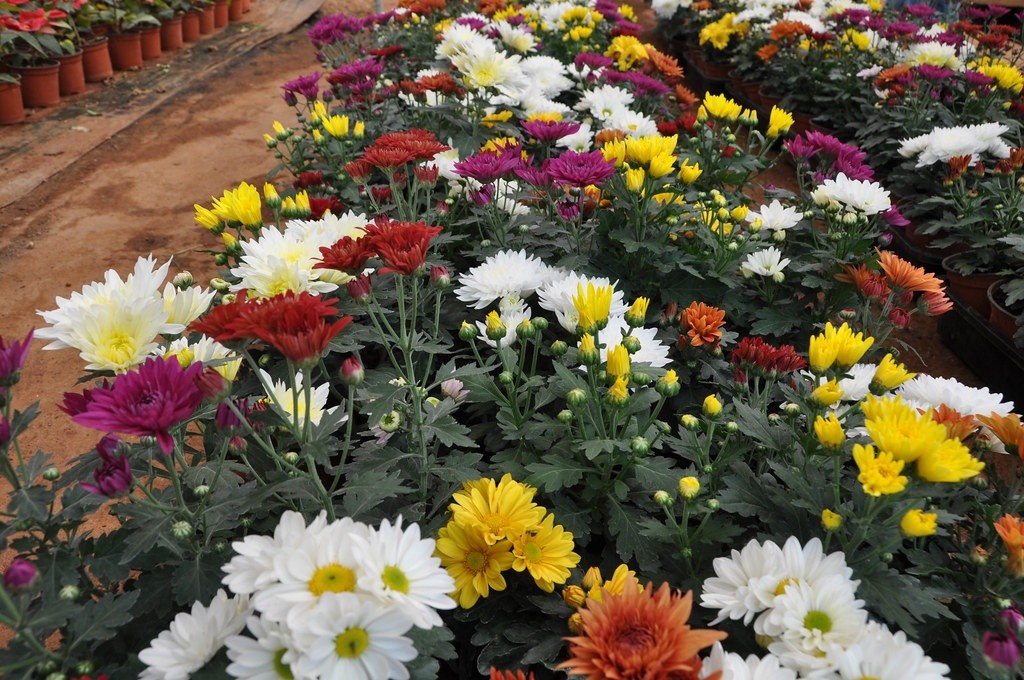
[0,1,1023,679]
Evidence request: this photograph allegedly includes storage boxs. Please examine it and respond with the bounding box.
[936,274,1024,407]
[888,225,946,300]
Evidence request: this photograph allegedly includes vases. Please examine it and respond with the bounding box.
[5,60,61,108]
[103,30,143,71]
[73,36,114,83]
[128,27,160,59]
[685,40,703,58]
[196,6,215,34]
[757,87,782,110]
[809,117,841,143]
[176,13,200,43]
[213,3,228,27]
[727,69,745,85]
[791,108,816,134]
[700,60,734,79]
[158,18,184,51]
[43,48,84,95]
[0,73,26,125]
[737,81,760,101]
[986,277,1022,342]
[943,249,1024,307]
